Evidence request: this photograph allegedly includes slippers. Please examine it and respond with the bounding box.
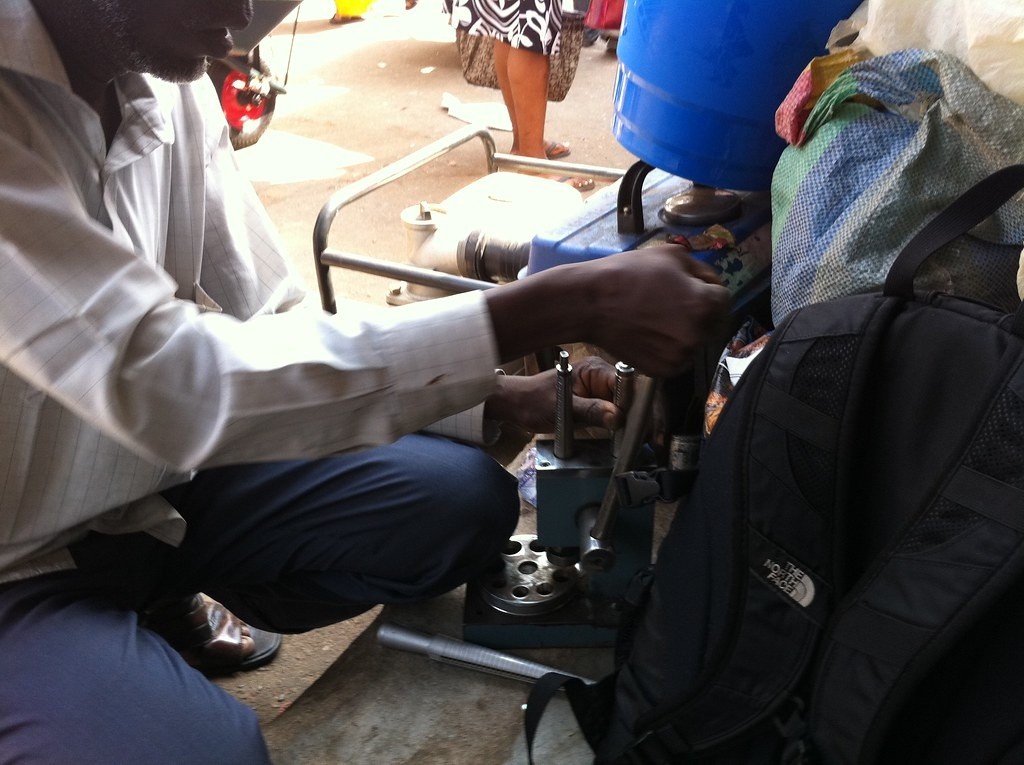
[558,176,595,192]
[545,142,571,160]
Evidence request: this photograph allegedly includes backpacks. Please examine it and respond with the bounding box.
[522,166,1024,765]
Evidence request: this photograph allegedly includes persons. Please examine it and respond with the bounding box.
[0,0,734,765]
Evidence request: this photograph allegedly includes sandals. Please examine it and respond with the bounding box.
[121,595,281,673]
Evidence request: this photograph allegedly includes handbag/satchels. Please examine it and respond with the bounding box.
[459,10,585,101]
